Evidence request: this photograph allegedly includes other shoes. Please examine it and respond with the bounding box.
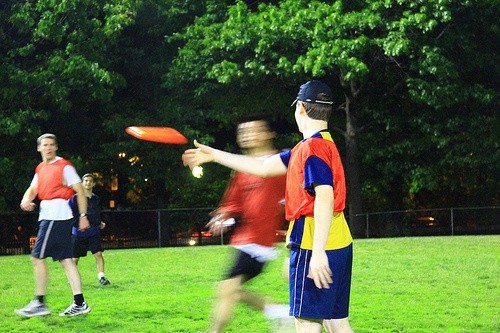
[99,277,110,285]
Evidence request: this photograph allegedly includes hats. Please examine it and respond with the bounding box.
[290,80,334,107]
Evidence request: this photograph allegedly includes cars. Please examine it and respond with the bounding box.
[409,210,451,236]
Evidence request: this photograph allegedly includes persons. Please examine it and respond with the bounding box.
[14,133,91,317]
[181,80,353,333]
[204,115,295,333]
[68,174,110,286]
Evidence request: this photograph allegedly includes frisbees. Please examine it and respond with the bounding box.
[125,124,188,146]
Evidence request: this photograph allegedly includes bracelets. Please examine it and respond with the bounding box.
[79,213,88,217]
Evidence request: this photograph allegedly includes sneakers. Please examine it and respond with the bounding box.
[14,299,50,317]
[59,302,91,317]
